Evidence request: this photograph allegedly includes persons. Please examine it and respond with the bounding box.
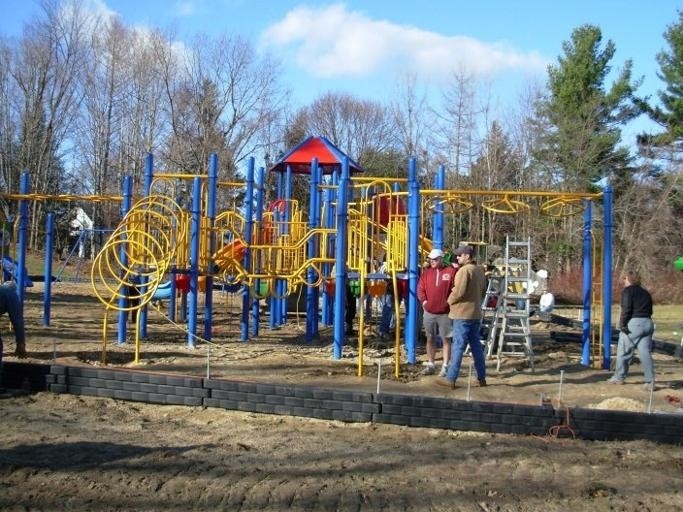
[364,251,402,339]
[432,246,487,389]
[415,247,456,376]
[328,261,356,335]
[605,268,658,392]
[0,277,28,367]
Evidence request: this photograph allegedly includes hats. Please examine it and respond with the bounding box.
[452,246,473,256]
[426,248,445,259]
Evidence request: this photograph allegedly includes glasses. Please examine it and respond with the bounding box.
[455,255,461,257]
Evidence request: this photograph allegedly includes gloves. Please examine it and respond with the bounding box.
[621,326,632,335]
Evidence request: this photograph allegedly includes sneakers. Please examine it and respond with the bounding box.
[421,367,487,391]
[605,377,656,392]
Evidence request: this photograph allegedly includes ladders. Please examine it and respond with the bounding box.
[463,234,533,372]
